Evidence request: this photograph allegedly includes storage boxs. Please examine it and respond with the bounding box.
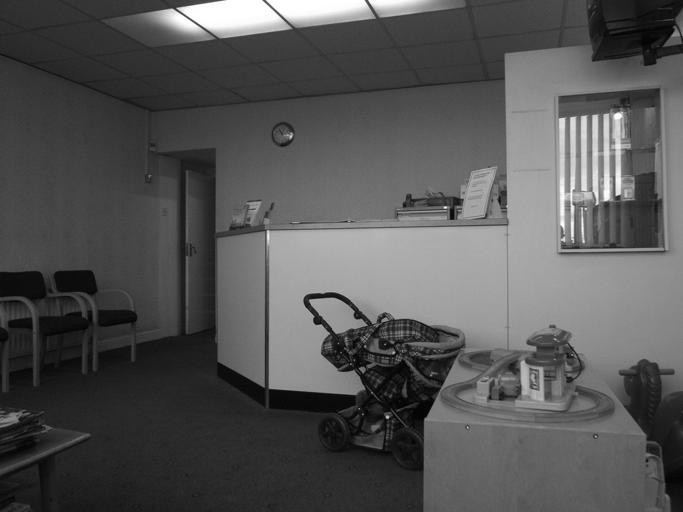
[620,172,654,200]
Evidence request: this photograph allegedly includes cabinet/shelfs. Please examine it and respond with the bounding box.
[584,199,660,248]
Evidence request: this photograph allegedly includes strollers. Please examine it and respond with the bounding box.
[302,292,466,470]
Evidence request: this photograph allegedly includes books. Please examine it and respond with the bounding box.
[0,401,52,457]
[396,204,508,222]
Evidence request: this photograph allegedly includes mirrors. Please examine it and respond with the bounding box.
[553,83,668,254]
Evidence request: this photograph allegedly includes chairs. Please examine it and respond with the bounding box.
[0,270,137,393]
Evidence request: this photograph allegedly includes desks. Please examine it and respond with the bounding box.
[0,429,91,511]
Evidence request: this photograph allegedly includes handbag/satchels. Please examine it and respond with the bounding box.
[321,312,439,372]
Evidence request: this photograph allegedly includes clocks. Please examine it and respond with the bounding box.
[271,122,293,146]
